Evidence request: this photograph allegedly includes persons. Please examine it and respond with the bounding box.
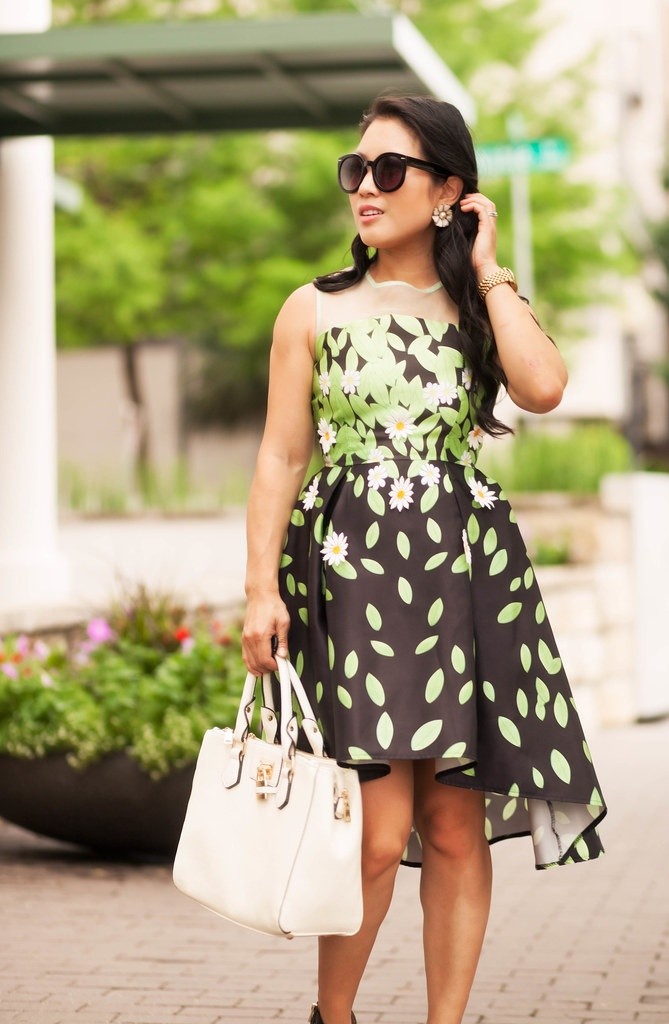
[242,97,608,1024]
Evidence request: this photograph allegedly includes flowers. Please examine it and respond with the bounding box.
[0,587,304,784]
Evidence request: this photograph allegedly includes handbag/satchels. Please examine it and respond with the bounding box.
[173,655,365,939]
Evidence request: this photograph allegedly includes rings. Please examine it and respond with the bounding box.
[488,211,498,217]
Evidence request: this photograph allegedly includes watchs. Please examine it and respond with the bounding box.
[478,266,518,301]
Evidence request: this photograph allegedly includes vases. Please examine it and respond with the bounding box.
[0,746,198,864]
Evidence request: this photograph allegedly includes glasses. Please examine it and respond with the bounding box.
[337,152,446,193]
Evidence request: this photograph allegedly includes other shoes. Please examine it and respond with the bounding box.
[308,1002,357,1024]
